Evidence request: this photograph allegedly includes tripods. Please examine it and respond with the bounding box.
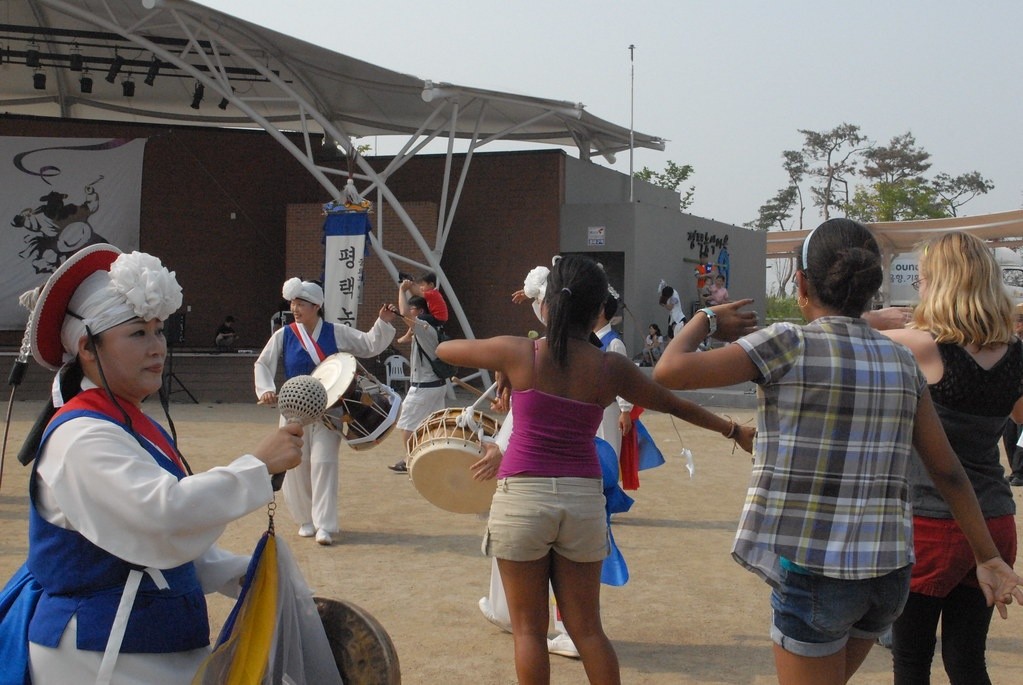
[140,345,200,406]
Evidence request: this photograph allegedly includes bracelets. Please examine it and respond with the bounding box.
[724,415,754,455]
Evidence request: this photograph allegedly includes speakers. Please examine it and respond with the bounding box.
[162,313,186,345]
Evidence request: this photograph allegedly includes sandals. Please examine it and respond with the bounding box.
[388,459,407,471]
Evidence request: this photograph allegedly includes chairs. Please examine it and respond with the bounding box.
[384,354,411,394]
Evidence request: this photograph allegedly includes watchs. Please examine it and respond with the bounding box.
[695,307,717,336]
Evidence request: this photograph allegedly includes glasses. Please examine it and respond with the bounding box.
[912,276,927,291]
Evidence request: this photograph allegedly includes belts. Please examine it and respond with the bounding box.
[411,379,446,387]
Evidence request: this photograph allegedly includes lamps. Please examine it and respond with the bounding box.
[190,83,207,110]
[106,53,126,82]
[26,49,40,67]
[218,85,235,109]
[122,79,136,97]
[69,53,83,70]
[34,72,47,90]
[80,78,92,94]
[144,59,164,86]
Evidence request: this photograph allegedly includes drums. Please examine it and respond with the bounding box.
[307,351,403,453]
[406,407,504,515]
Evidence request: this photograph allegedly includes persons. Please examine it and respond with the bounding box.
[651,218,1023,684]
[213,316,240,349]
[856,230,1023,685]
[0,242,305,684]
[1002,302,1023,485]
[642,323,664,365]
[388,278,447,472]
[253,276,398,544]
[435,254,759,685]
[397,270,448,344]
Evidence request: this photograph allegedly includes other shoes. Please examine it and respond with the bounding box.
[547,633,580,657]
[297,523,332,544]
[1005,474,1023,486]
[479,596,513,634]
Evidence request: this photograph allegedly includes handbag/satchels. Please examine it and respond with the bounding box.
[432,328,458,378]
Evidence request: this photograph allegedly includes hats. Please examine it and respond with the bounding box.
[21,240,124,370]
